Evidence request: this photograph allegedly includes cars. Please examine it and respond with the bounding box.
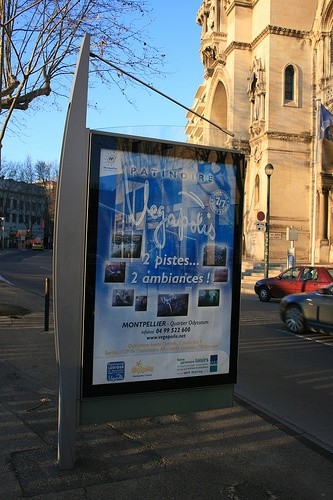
[280,282,333,334]
[32,242,44,251]
[254,266,333,303]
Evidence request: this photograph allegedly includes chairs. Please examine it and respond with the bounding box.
[306,273,312,279]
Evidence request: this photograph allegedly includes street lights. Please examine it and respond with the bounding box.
[0,214,7,250]
[264,163,274,279]
